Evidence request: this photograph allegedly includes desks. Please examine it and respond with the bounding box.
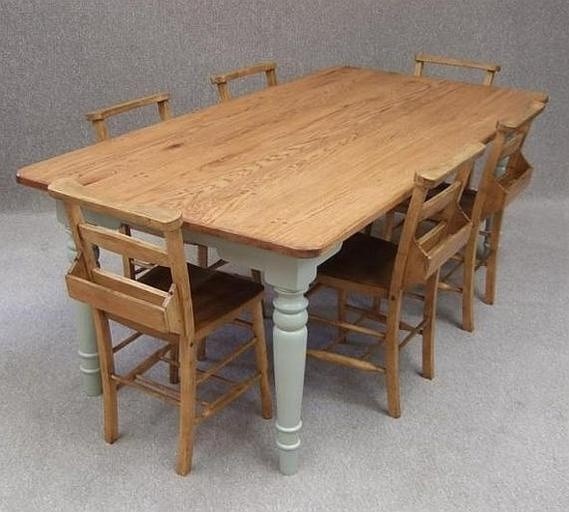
[18,68,550,477]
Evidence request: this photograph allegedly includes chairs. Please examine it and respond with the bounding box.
[214,62,276,101]
[85,92,266,322]
[385,98,551,329]
[44,177,274,476]
[414,53,501,87]
[307,143,488,420]
